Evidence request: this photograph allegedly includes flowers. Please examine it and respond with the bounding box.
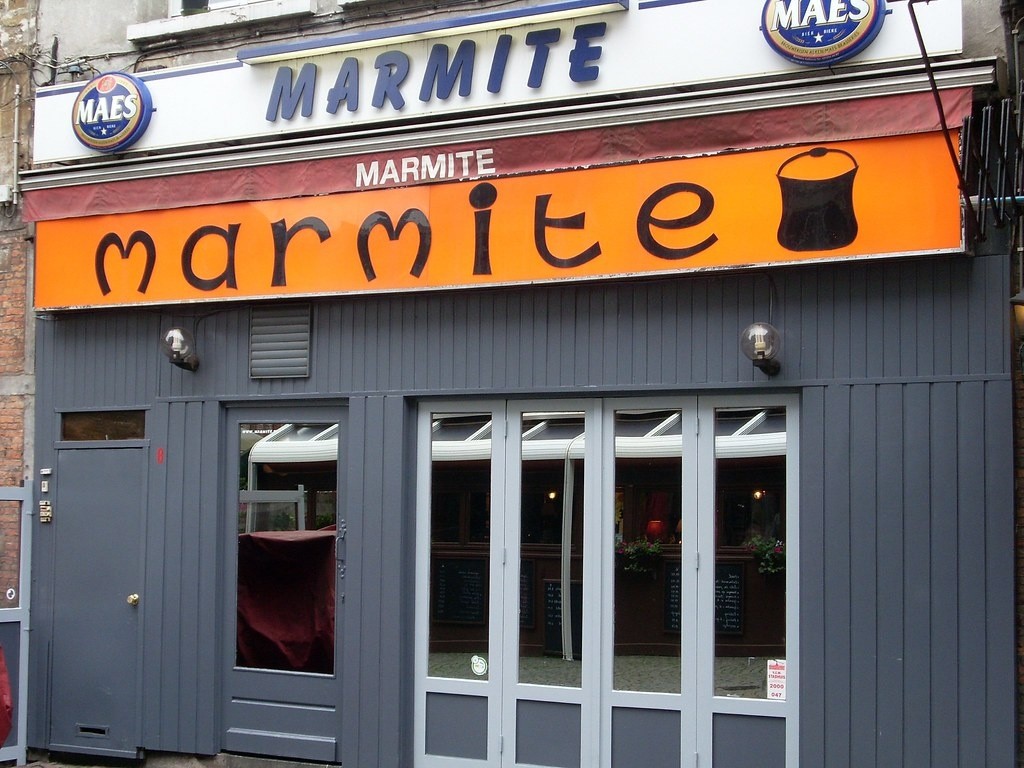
[616,538,661,570]
[745,535,787,573]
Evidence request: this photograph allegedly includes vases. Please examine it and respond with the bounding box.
[618,570,655,590]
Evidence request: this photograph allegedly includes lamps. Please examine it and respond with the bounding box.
[739,324,782,377]
[162,328,201,374]
